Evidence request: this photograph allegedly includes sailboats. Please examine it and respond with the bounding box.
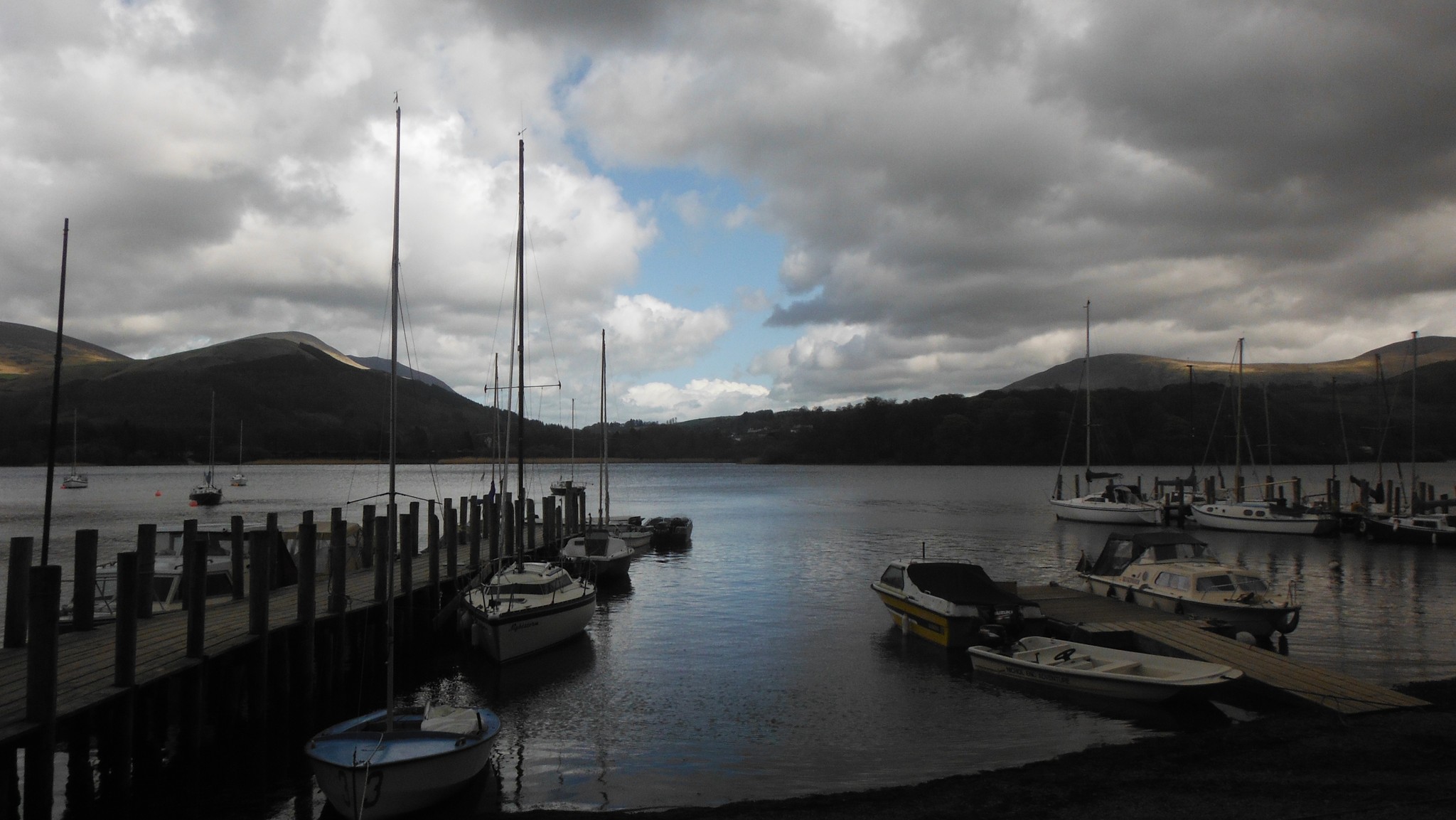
[1049,299,1167,525]
[230,419,248,487]
[304,94,500,819]
[63,407,89,488]
[1162,337,1343,535]
[560,327,633,583]
[188,390,225,505]
[1341,330,1456,541]
[455,127,598,666]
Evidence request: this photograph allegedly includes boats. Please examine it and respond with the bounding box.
[1075,531,1302,641]
[870,541,1245,707]
[53,522,367,628]
[602,513,696,550]
